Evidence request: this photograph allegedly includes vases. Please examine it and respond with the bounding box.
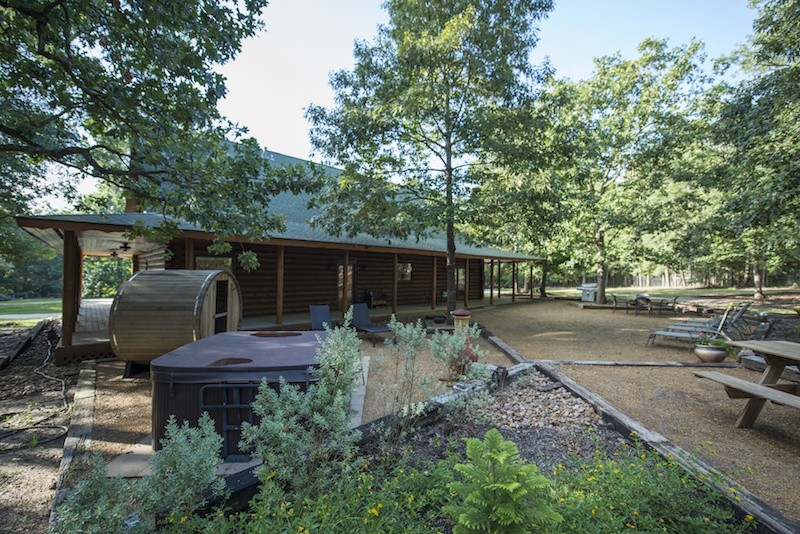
[694,344,728,362]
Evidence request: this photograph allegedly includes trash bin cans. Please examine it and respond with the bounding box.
[582,283,597,302]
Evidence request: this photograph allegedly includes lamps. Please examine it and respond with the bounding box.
[111,252,118,257]
[120,242,131,251]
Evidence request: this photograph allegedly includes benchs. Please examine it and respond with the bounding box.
[742,356,800,383]
[693,370,800,410]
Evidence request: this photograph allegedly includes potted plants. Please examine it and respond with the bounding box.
[432,309,446,323]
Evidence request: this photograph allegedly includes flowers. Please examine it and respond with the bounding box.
[689,332,735,357]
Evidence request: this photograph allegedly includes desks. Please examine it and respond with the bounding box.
[725,340,800,427]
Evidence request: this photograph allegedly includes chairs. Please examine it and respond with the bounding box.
[611,295,754,351]
[310,304,333,330]
[423,288,444,306]
[367,287,387,308]
[349,302,397,347]
